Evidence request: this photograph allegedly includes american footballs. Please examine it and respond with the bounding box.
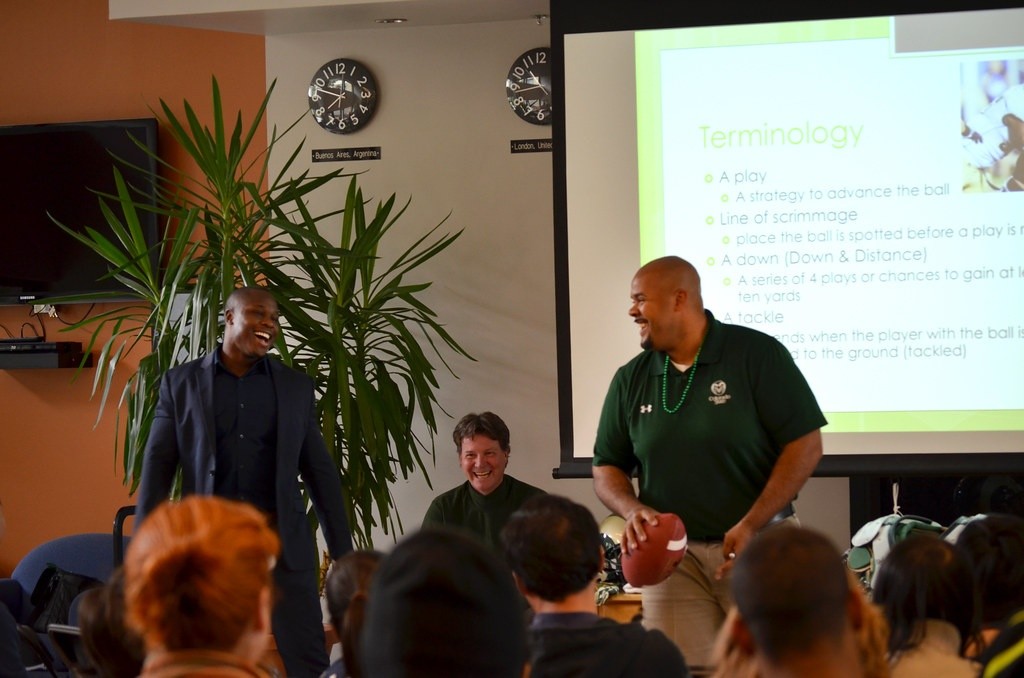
[622,513,688,588]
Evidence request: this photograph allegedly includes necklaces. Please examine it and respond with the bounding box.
[662,342,703,412]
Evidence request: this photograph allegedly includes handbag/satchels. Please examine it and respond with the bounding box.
[26,564,107,633]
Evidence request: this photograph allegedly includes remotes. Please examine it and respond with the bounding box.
[0,334,44,344]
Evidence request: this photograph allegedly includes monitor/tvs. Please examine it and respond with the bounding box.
[0,119,166,307]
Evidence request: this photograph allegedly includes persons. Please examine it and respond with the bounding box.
[317,495,688,678]
[419,410,548,548]
[140,293,350,678]
[79,496,282,678]
[713,512,1024,678]
[588,255,827,666]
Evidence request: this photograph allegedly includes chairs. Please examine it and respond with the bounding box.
[0,535,135,678]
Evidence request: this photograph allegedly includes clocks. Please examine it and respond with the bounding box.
[307,58,377,134]
[505,46,553,124]
